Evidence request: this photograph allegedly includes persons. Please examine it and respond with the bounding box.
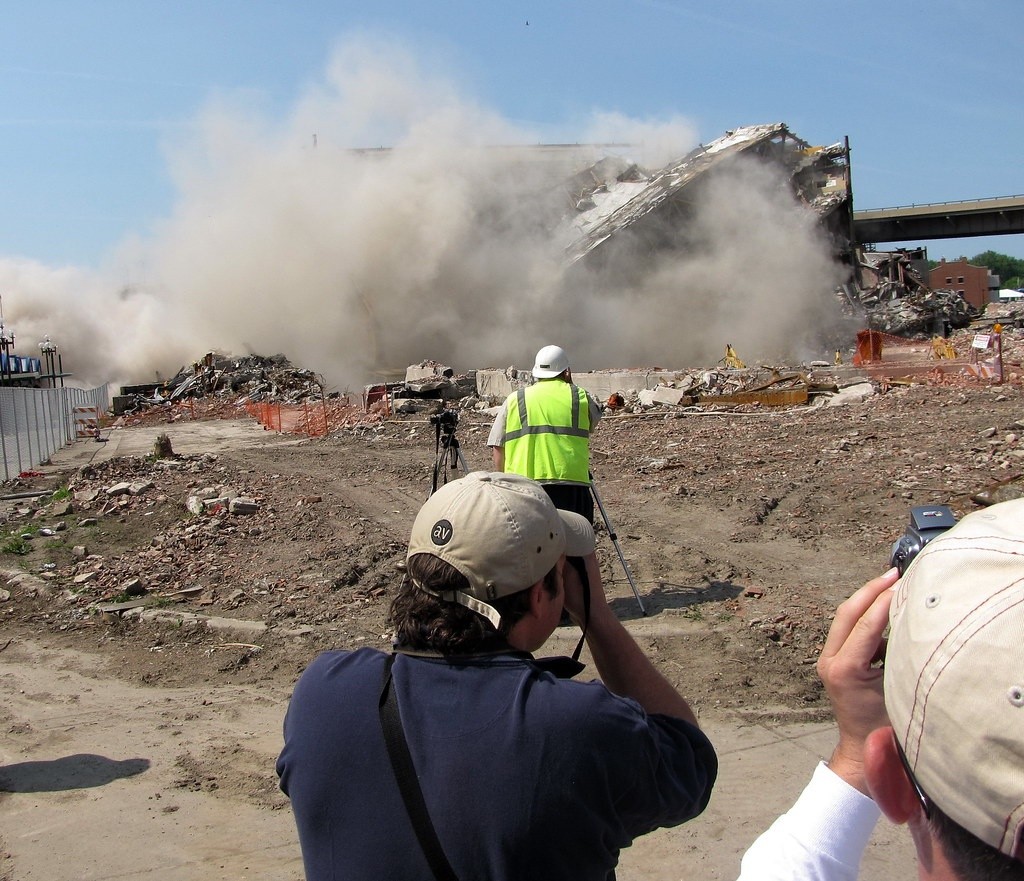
[485,345,602,627]
[275,470,719,881]
[734,497,1024,881]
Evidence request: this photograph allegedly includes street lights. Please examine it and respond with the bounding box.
[0,325,16,386]
[38,334,59,388]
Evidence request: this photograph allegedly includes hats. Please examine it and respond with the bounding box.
[880,497,1023,860]
[402,469,598,629]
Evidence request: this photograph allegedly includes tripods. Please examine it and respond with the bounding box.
[427,424,469,501]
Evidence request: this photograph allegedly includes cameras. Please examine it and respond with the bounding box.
[888,504,956,579]
[431,409,459,426]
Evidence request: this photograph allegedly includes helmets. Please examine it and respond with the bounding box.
[530,345,571,379]
